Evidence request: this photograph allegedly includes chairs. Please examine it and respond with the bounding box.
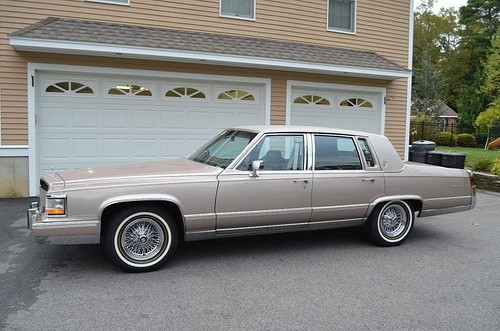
[261,150,286,169]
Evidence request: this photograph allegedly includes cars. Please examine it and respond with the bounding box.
[27,125,477,274]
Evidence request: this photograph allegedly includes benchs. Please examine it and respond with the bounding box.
[319,150,362,171]
